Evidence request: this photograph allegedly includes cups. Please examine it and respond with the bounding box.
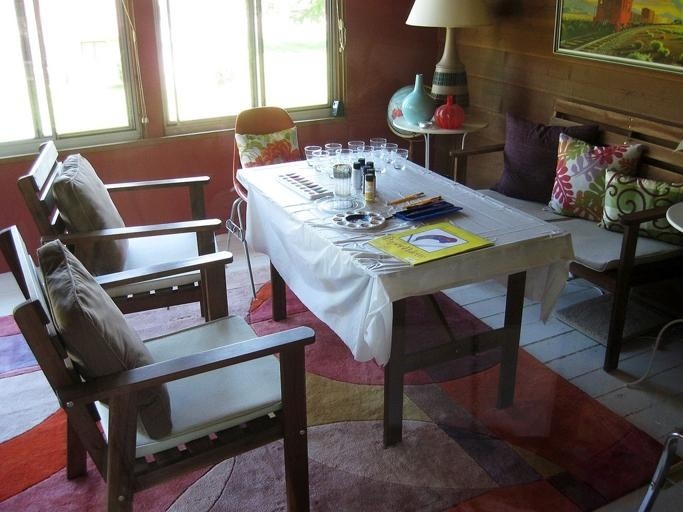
[304,136,408,178]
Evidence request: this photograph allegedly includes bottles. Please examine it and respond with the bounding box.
[351,157,377,202]
[332,164,352,205]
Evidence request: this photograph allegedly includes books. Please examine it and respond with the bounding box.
[368,222,496,269]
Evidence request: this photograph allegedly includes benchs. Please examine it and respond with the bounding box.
[449,97,683,372]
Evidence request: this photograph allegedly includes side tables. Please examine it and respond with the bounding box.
[619,202,682,386]
[393,114,488,179]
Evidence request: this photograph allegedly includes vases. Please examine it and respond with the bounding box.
[402,73,436,126]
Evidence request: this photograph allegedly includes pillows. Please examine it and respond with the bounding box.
[542,132,644,221]
[597,169,683,245]
[235,125,301,168]
[489,112,599,203]
[52,154,130,275]
[36,238,173,440]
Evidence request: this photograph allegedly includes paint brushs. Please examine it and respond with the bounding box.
[387,192,424,205]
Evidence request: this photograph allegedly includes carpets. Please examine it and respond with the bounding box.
[0,265,683,512]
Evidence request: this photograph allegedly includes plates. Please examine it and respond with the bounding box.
[332,210,386,229]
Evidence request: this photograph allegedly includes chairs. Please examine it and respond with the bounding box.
[0,225,317,512]
[225,107,301,299]
[18,141,222,318]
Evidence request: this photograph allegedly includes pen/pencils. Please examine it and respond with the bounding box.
[401,195,442,209]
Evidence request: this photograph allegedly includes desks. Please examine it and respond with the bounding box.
[237,149,572,449]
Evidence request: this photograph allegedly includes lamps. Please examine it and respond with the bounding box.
[405,0,499,108]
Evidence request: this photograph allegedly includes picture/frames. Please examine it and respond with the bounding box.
[552,0,683,75]
